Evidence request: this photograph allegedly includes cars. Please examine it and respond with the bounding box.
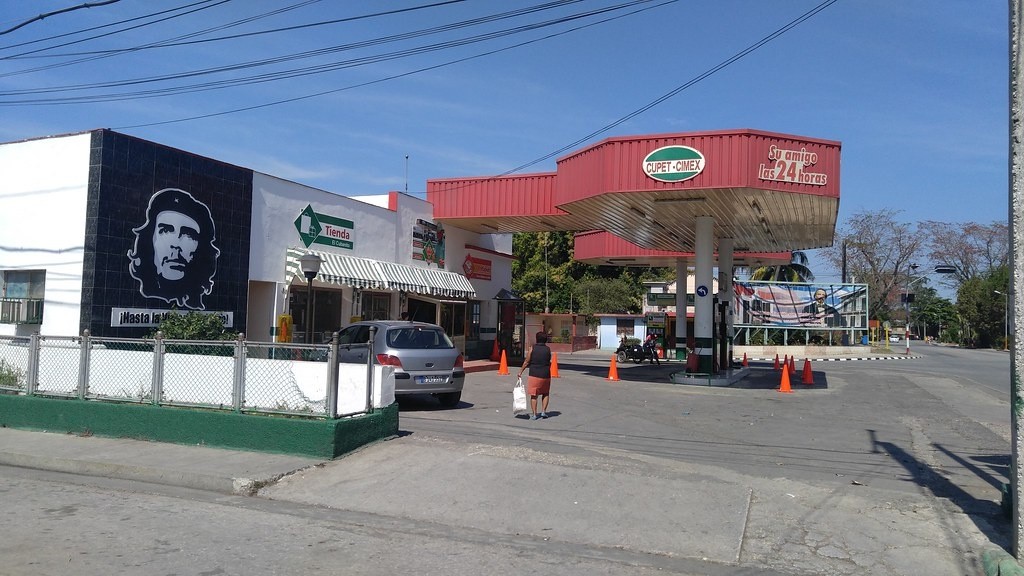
[889,334,900,343]
[306,319,466,409]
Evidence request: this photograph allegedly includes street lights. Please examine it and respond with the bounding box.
[296,250,326,361]
[906,262,919,340]
[994,290,1010,351]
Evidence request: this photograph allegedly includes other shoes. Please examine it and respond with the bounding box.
[541,412,548,418]
[532,416,538,420]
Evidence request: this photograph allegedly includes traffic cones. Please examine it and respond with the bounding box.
[782,355,789,371]
[742,352,749,367]
[800,357,809,379]
[549,352,561,378]
[801,361,816,384]
[491,338,500,361]
[495,349,510,376]
[777,361,796,393]
[604,354,621,381]
[772,354,781,372]
[788,355,797,374]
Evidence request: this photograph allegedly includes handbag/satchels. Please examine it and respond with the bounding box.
[513,377,527,415]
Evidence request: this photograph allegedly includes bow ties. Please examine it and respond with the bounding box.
[817,304,825,308]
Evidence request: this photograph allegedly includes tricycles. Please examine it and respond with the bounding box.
[616,337,661,366]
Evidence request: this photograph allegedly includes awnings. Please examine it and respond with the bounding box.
[282,247,476,313]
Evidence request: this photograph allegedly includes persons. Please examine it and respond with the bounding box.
[518,332,550,419]
[643,334,661,366]
[801,288,846,326]
